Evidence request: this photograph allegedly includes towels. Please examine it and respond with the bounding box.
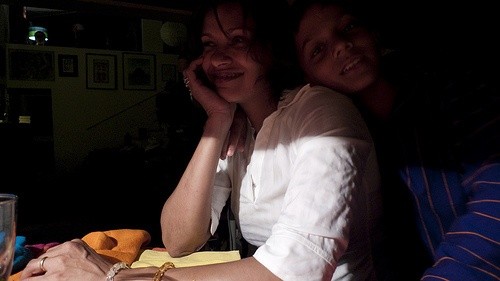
[0,228,150,281]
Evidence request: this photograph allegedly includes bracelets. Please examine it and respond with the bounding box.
[103,261,131,281]
[152,262,176,281]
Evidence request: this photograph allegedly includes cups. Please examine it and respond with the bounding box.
[0,193,18,281]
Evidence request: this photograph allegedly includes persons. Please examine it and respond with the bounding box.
[19,0,385,281]
[290,0,500,281]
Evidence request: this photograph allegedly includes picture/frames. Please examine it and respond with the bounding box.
[161,63,177,83]
[8,49,55,81]
[58,54,78,77]
[122,52,157,91]
[86,52,117,91]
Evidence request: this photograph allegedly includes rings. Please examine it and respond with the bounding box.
[182,76,190,85]
[40,256,47,272]
